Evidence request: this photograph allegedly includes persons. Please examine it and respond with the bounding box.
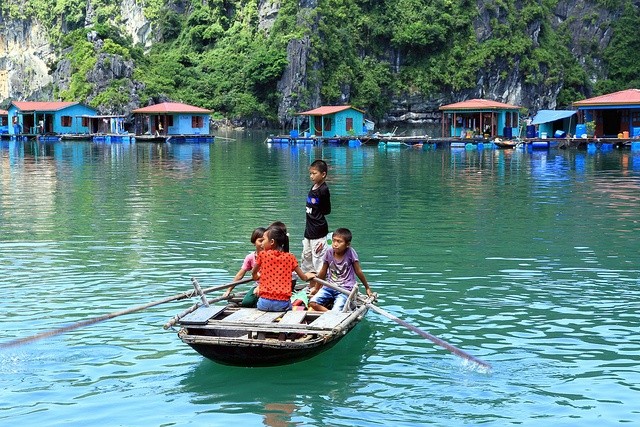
[300,160,332,294]
[222,227,273,307]
[307,228,377,313]
[251,226,316,312]
[269,221,298,292]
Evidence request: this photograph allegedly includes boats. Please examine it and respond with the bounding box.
[494,140,518,148]
[55,134,94,140]
[163,277,378,368]
[340,138,381,147]
[132,130,172,143]
